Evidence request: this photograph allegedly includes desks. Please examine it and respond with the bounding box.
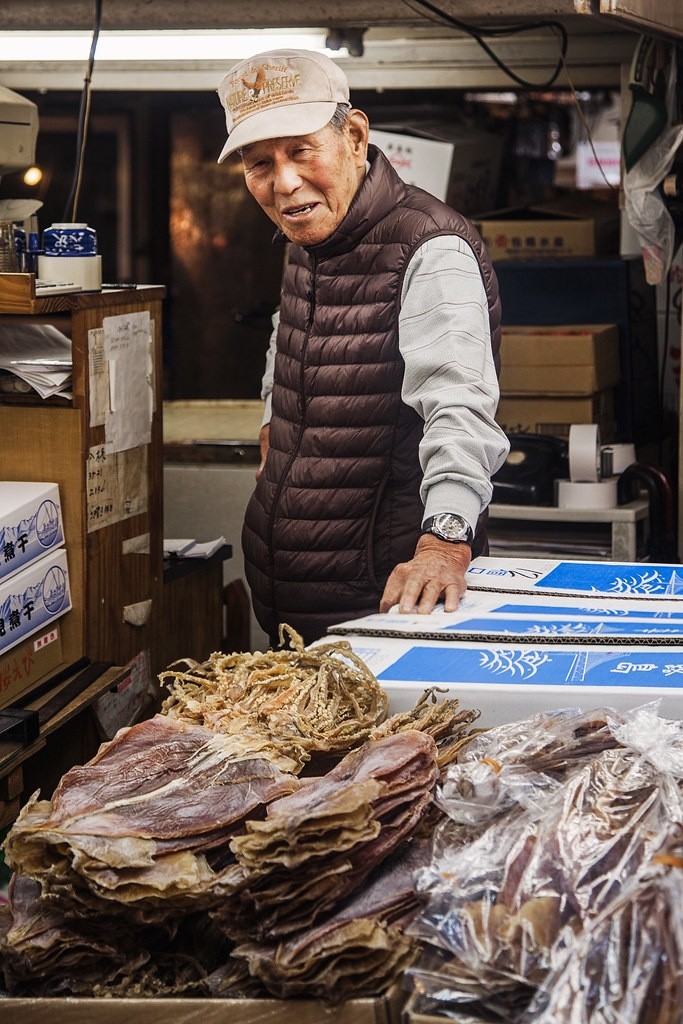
[489,490,649,563]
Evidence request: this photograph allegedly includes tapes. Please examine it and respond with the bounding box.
[38,256,101,291]
[568,423,601,484]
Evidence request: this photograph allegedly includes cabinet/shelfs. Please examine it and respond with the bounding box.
[0,270,232,839]
[493,255,662,448]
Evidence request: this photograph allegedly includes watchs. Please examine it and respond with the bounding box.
[423,513,473,547]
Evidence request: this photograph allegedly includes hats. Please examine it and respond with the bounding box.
[216,50,350,164]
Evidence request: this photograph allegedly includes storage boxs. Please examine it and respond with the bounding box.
[0,480,65,584]
[0,548,74,656]
[312,556,683,736]
[495,393,613,444]
[470,195,620,261]
[0,954,457,1024]
[0,619,65,706]
[499,325,620,397]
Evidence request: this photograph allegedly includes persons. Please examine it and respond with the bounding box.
[217,50,510,653]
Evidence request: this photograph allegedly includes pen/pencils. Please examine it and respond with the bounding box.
[101,283,138,289]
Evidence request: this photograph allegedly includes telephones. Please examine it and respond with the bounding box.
[491,433,569,508]
